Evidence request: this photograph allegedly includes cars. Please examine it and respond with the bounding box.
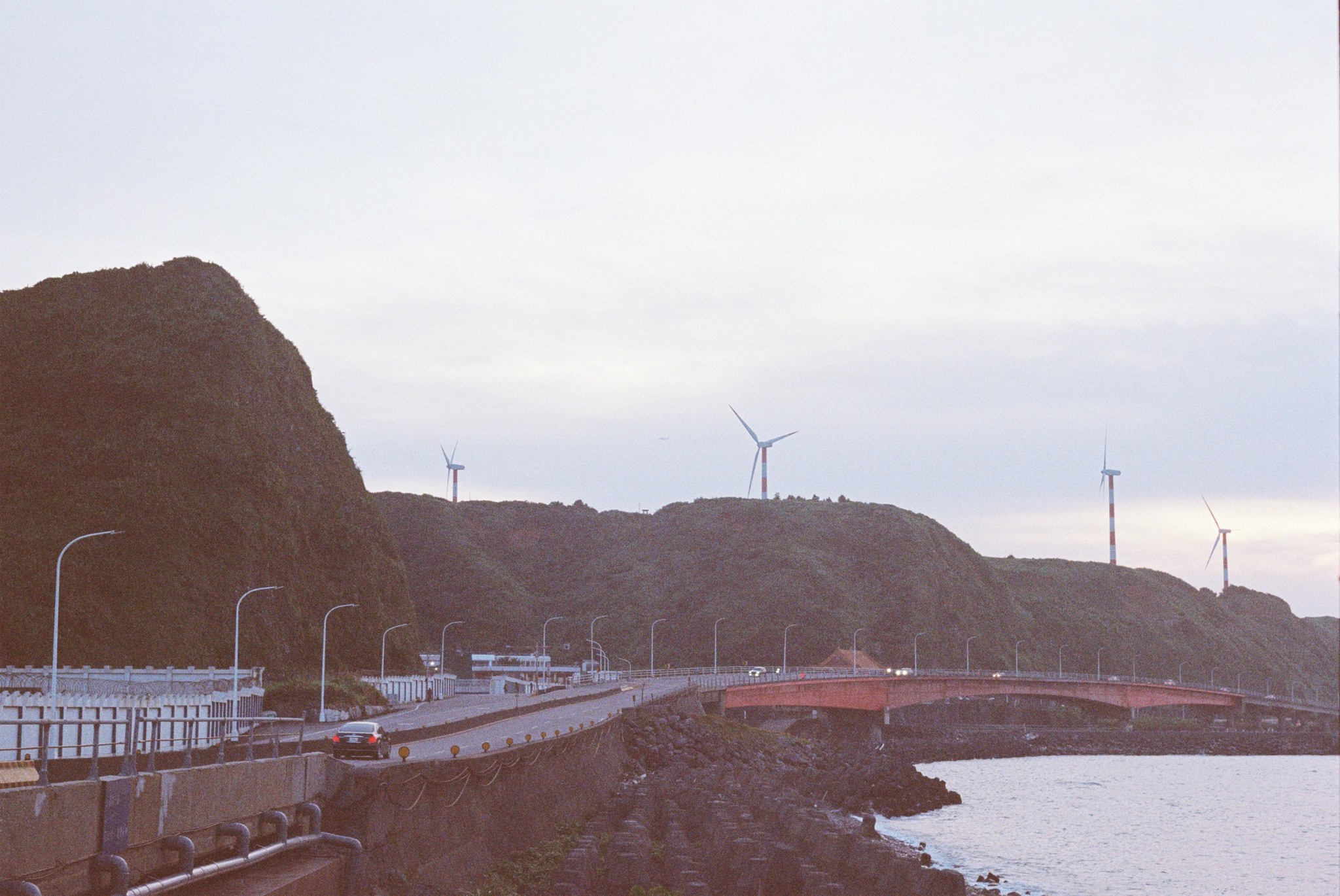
[1265,695,1276,700]
[1107,675,1121,682]
[895,668,913,677]
[748,666,767,678]
[1220,687,1229,692]
[992,672,1005,678]
[332,721,392,760]
[1164,679,1175,686]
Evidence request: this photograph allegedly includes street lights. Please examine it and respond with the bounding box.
[853,628,866,674]
[914,632,926,676]
[589,615,610,682]
[714,617,725,673]
[230,585,286,743]
[651,619,667,678]
[381,623,412,700]
[542,616,564,693]
[438,620,467,700]
[1016,640,1025,674]
[1098,647,1106,681]
[319,602,361,724]
[1059,645,1069,678]
[1179,661,1188,686]
[1132,654,1142,683]
[49,528,127,754]
[967,636,978,672]
[1211,666,1220,689]
[783,624,798,673]
[619,658,631,681]
